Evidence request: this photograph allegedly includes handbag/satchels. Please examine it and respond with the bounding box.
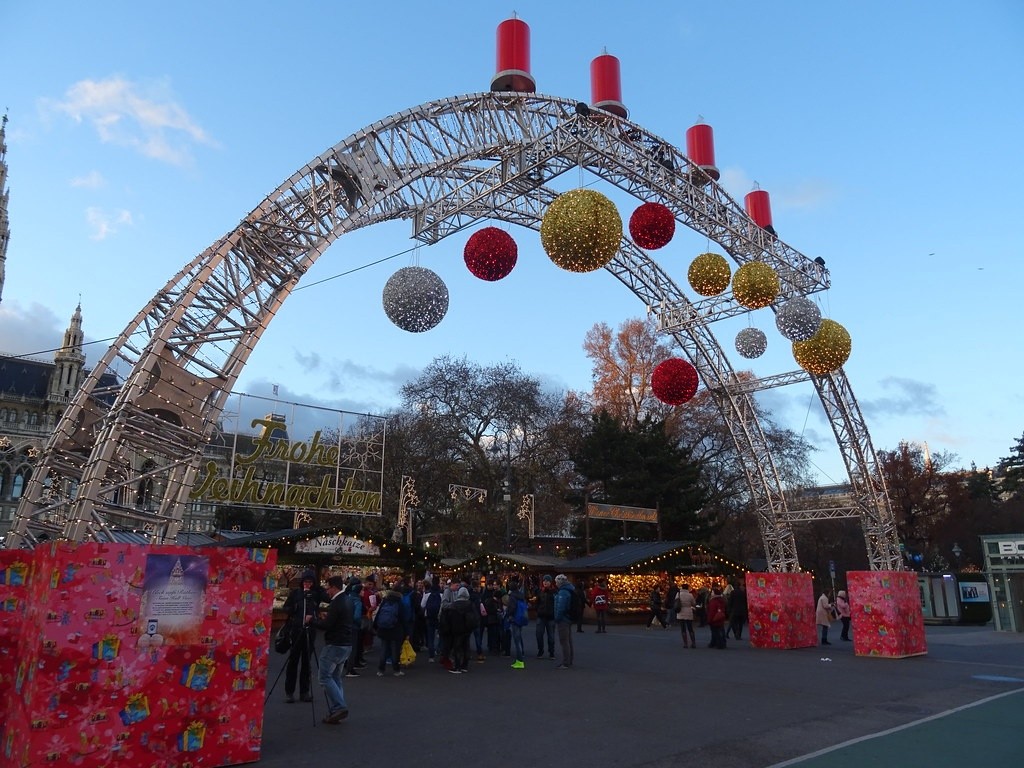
[274,619,295,655]
[399,640,417,666]
[671,592,682,613]
[714,609,725,627]
[594,595,606,605]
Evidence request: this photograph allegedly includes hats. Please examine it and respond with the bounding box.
[555,574,567,585]
[458,587,470,598]
[501,594,510,605]
[542,573,549,578]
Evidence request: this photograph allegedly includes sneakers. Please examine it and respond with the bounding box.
[510,660,525,670]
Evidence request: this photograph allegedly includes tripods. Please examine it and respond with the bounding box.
[265,623,331,726]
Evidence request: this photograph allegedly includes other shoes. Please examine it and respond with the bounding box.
[323,708,349,724]
[375,671,384,678]
[344,656,369,680]
[393,670,405,678]
[283,692,313,704]
[428,656,470,675]
[556,664,570,670]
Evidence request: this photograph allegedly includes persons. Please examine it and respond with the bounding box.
[307,577,355,723]
[589,579,609,633]
[815,590,836,645]
[282,571,330,703]
[448,587,478,673]
[507,581,528,658]
[535,574,557,660]
[553,574,576,669]
[576,584,589,633]
[836,591,852,641]
[372,583,410,677]
[665,581,747,649]
[343,573,510,677]
[675,584,696,648]
[646,585,670,630]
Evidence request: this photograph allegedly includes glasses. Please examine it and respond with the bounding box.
[326,585,334,592]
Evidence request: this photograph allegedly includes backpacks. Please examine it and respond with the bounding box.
[510,596,529,626]
[399,589,415,624]
[558,588,579,623]
[376,598,399,629]
